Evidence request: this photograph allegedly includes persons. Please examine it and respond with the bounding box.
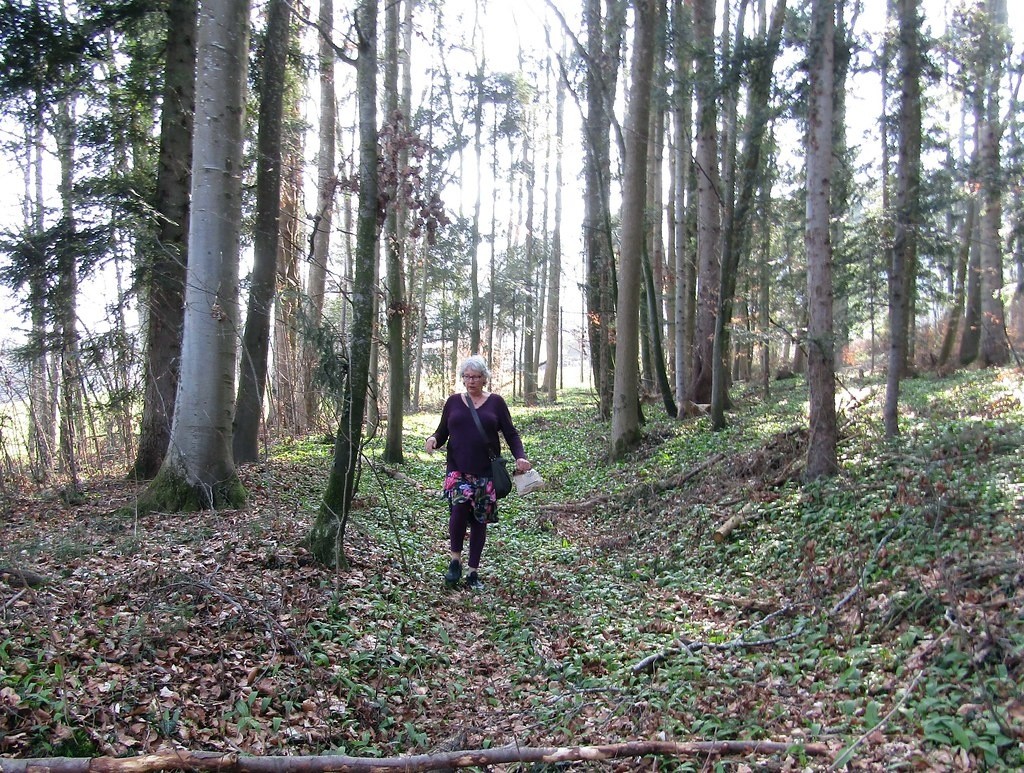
[424,354,534,585]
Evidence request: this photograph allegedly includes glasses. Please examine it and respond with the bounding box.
[463,374,483,381]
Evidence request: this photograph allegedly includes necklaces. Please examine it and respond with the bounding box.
[468,392,484,404]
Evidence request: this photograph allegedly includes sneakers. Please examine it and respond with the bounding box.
[445,560,462,582]
[466,570,485,589]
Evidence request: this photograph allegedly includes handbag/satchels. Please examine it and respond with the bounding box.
[513,462,544,496]
[491,458,512,499]
